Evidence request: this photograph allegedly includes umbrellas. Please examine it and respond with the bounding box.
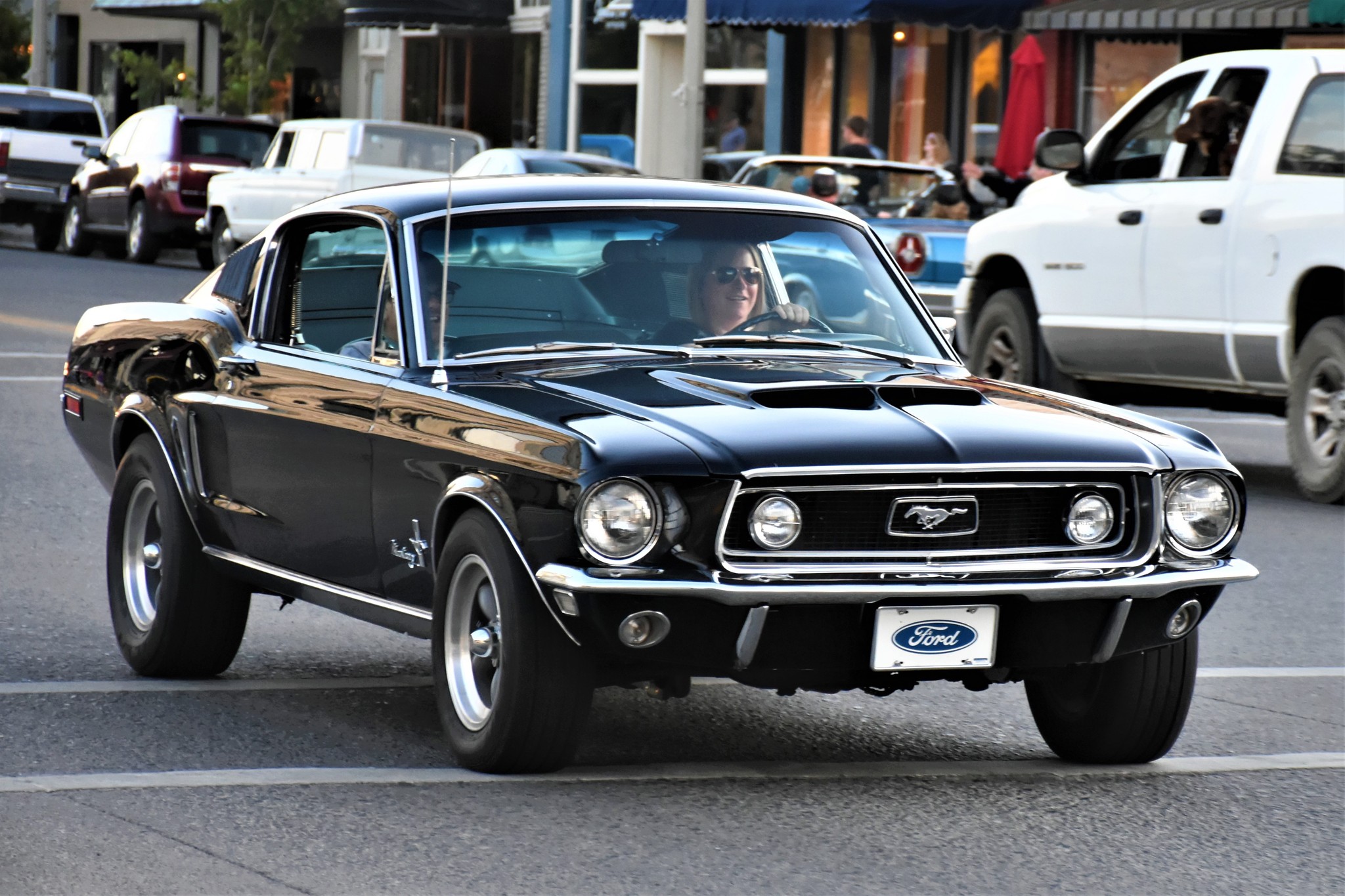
[993,35,1046,178]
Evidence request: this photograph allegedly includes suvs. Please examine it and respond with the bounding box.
[197,117,490,271]
[0,85,111,246]
[943,45,1345,502]
[62,105,289,264]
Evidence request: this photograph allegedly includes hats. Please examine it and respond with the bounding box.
[721,111,738,122]
[378,250,462,300]
[849,116,870,137]
[813,167,837,188]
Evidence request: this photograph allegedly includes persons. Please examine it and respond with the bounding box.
[1174,96,1243,176]
[836,115,879,200]
[918,130,950,168]
[341,252,462,363]
[962,125,1057,208]
[810,166,838,204]
[719,113,746,151]
[651,238,817,345]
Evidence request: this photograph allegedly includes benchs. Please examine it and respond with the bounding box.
[452,323,653,354]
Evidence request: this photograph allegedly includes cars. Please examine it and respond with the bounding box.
[60,174,1260,773]
[458,145,680,268]
[615,155,997,322]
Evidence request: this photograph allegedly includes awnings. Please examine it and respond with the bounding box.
[855,0,1043,34]
[631,1,867,28]
[1024,1,1309,29]
[1309,0,1345,28]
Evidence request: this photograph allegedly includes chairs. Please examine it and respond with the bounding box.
[903,181,968,217]
[838,201,872,219]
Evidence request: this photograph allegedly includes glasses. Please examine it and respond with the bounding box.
[422,285,456,306]
[708,265,764,285]
